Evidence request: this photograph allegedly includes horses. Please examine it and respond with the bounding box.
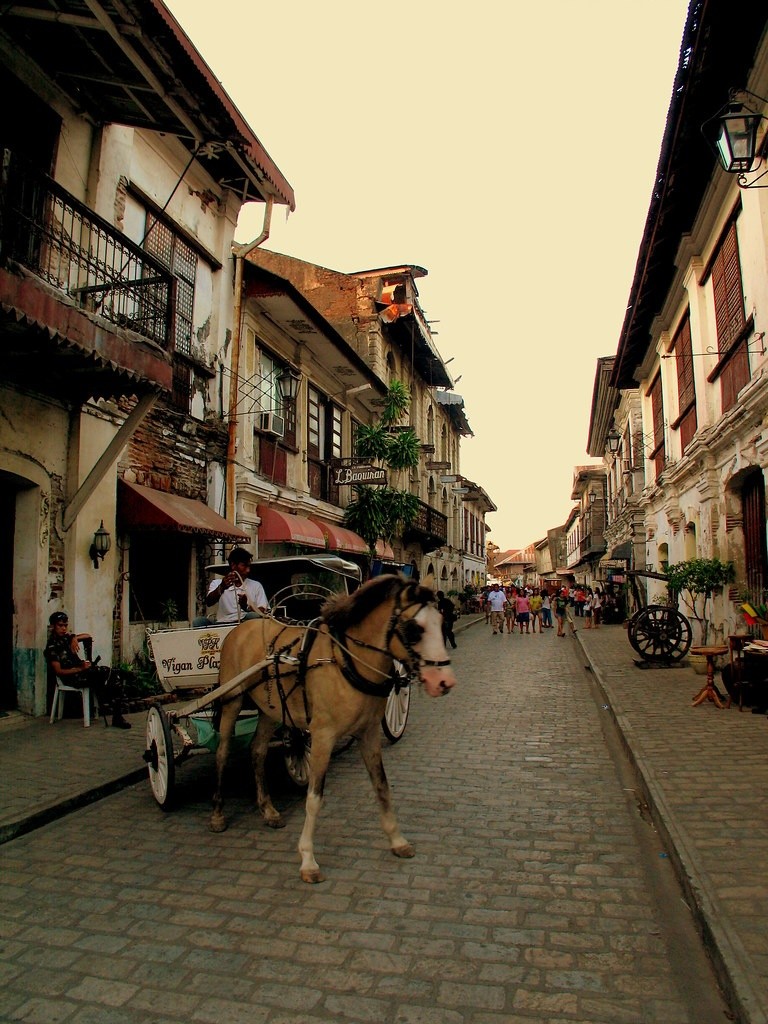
[204,573,457,884]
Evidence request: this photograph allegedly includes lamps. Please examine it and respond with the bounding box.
[659,559,669,568]
[220,359,303,420]
[604,423,656,459]
[645,563,653,572]
[89,520,112,568]
[587,487,624,509]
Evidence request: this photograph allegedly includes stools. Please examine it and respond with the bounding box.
[688,633,754,712]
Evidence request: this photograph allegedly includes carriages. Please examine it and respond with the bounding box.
[138,552,461,885]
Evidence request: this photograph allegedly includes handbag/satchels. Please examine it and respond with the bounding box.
[513,608,516,612]
[583,601,590,611]
[529,612,534,620]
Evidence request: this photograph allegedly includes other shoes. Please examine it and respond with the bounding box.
[551,626,554,628]
[539,631,544,634]
[533,630,536,633]
[508,632,511,634]
[584,624,591,629]
[500,630,503,633]
[520,631,523,634]
[112,716,131,729]
[562,632,565,637]
[493,631,497,635]
[545,625,548,628]
[525,631,530,634]
[592,624,596,629]
[511,629,514,634]
[557,634,561,636]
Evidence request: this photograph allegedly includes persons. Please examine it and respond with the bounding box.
[192,548,271,627]
[43,612,132,729]
[437,584,601,650]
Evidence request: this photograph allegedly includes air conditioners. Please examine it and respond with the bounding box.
[261,412,284,437]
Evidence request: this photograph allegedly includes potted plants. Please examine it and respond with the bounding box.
[447,584,477,619]
[661,556,735,676]
[622,584,633,629]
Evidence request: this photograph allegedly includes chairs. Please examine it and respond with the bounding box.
[48,675,99,728]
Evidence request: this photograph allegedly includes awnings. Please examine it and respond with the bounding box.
[257,504,395,560]
[118,479,251,544]
[0,256,172,531]
[600,541,630,560]
[377,300,474,437]
[0,0,295,213]
